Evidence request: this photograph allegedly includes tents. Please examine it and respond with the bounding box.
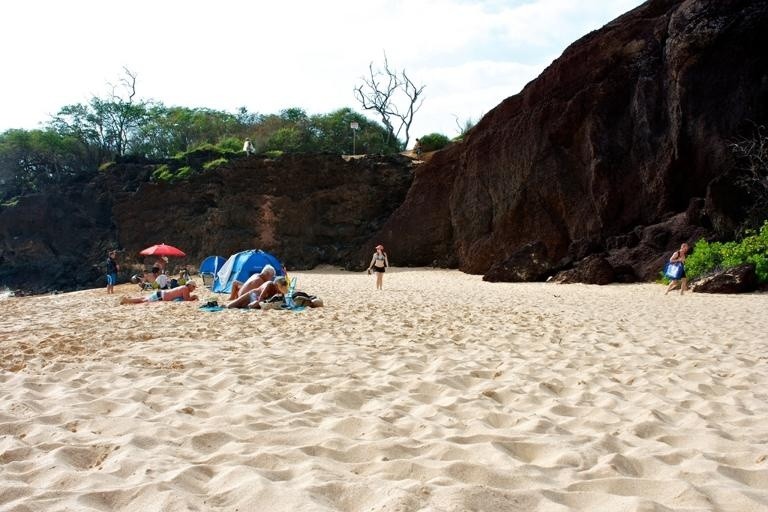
[212,248,286,294]
[199,256,227,278]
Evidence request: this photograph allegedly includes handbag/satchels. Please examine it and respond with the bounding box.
[666,263,683,279]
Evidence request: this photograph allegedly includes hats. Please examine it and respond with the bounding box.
[376,245,383,250]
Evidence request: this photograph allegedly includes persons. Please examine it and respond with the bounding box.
[119,280,199,304]
[367,245,389,290]
[225,264,288,309]
[107,251,120,294]
[137,256,191,289]
[664,243,689,295]
[243,138,256,153]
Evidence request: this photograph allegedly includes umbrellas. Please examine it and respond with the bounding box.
[139,243,187,274]
